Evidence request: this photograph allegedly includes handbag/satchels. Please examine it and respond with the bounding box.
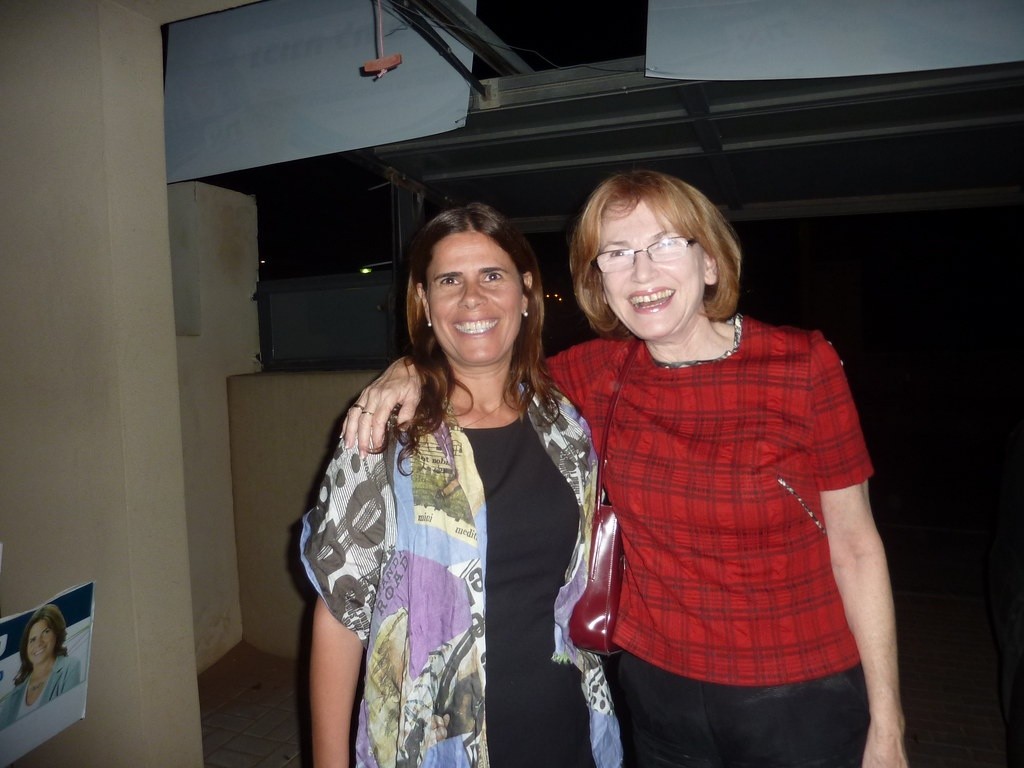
[567,503,626,658]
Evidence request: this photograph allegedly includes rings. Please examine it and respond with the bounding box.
[353,404,364,410]
[363,411,373,415]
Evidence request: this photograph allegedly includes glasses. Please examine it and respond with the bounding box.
[591,235,698,274]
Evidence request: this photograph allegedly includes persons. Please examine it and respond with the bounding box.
[338,169,909,768]
[0,604,81,729]
[299,202,627,768]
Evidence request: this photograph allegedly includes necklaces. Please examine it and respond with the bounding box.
[27,675,45,690]
[656,311,743,369]
